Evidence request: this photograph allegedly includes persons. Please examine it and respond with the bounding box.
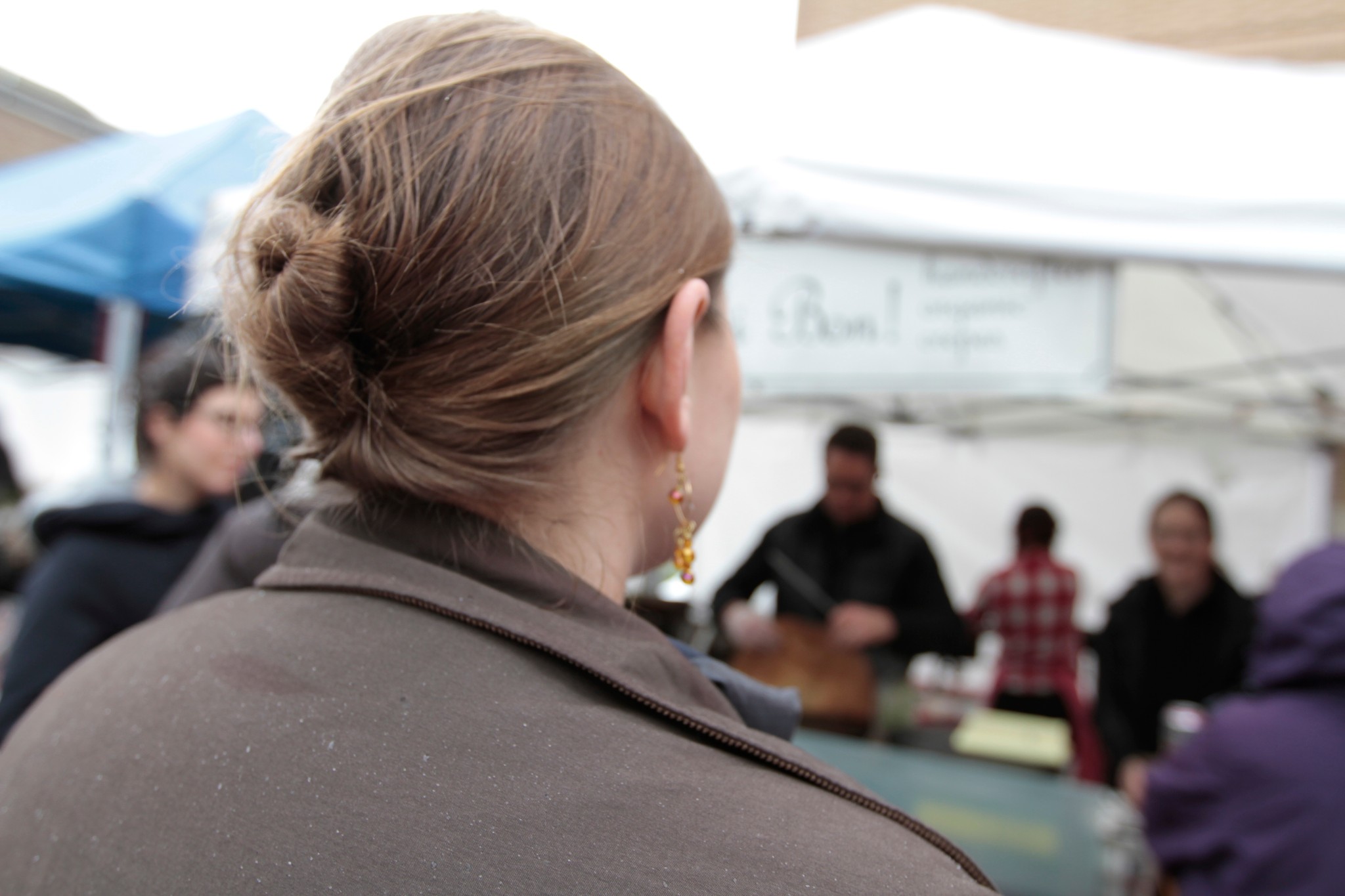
[712,422,978,685]
[1141,536,1345,894]
[0,9,1017,896]
[1094,487,1269,802]
[970,502,1108,788]
[2,325,267,743]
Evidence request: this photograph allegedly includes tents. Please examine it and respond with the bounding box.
[1,106,306,492]
[632,8,1345,570]
